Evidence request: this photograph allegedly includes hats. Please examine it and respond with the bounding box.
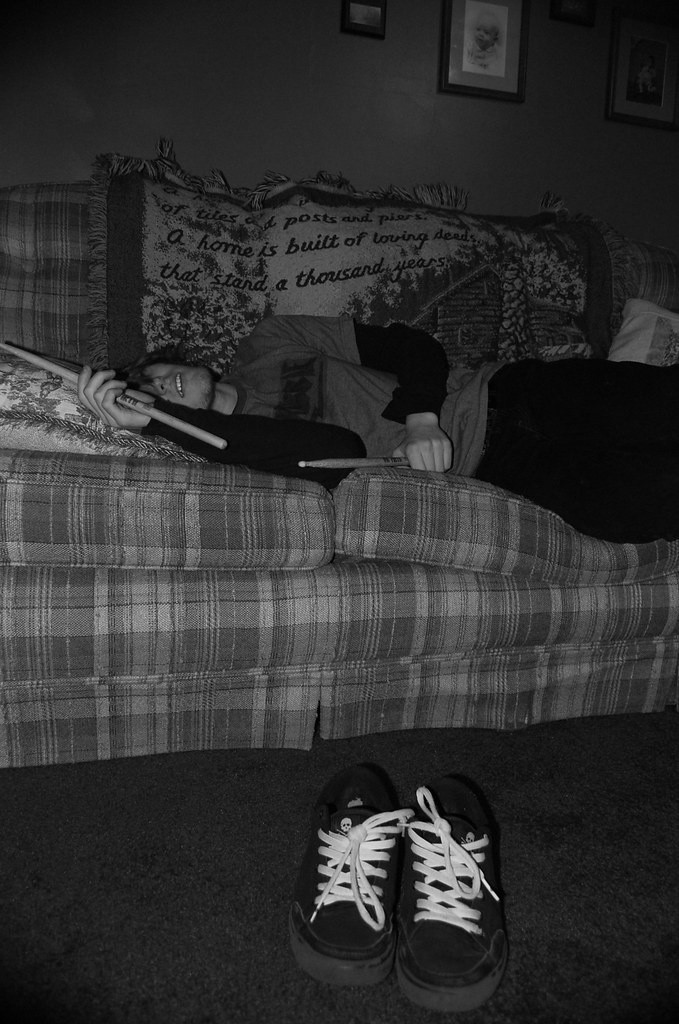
[639,54,655,68]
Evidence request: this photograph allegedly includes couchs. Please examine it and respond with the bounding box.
[0,137,678,770]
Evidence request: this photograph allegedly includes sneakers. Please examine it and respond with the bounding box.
[394,776,508,1012]
[288,764,415,988]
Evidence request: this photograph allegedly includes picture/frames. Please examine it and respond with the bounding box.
[549,0,597,25]
[438,0,531,103]
[342,0,387,41]
[604,8,679,131]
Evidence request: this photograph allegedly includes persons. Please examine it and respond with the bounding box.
[78,315,679,544]
[467,13,504,69]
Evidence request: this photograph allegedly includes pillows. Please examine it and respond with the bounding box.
[0,353,204,462]
[608,299,679,367]
